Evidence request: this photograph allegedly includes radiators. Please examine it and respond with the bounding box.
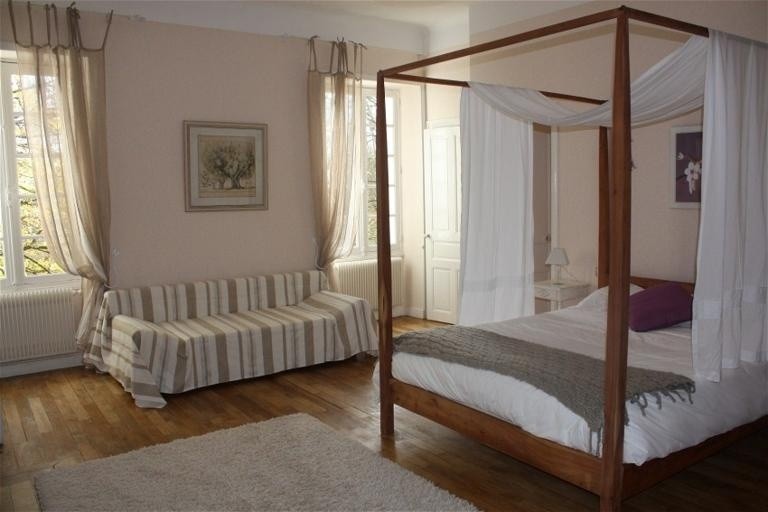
[0,287,94,364]
[333,256,403,311]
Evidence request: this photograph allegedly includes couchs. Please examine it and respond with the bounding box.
[82,270,380,410]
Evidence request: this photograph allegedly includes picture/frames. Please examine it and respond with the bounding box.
[669,126,702,211]
[182,118,268,213]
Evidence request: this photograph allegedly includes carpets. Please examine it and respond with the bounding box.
[33,414,476,512]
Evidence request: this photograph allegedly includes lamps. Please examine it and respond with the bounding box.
[545,248,568,284]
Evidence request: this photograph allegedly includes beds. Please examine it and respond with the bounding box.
[375,6,768,512]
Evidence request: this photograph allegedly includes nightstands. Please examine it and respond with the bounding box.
[535,279,588,312]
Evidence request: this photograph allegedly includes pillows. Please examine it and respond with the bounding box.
[628,285,692,332]
